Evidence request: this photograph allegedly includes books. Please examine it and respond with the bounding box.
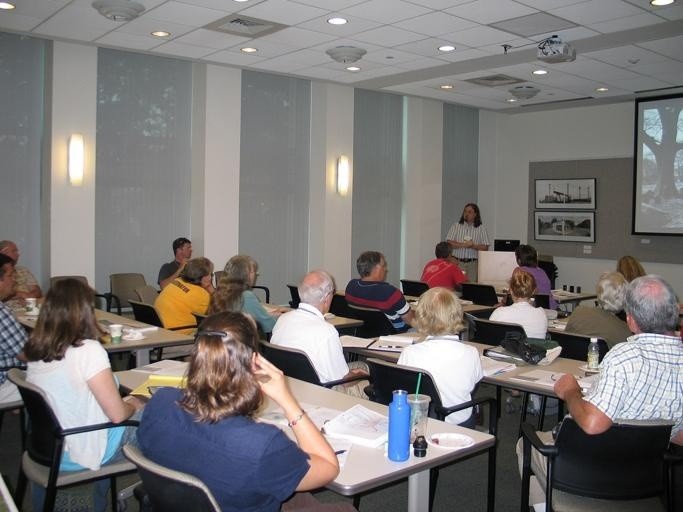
[257,400,343,433]
[327,438,352,466]
[130,375,187,397]
[131,358,191,377]
[95,317,158,331]
[510,370,565,386]
[549,321,566,331]
[552,289,583,298]
[339,335,376,350]
[479,355,516,377]
[368,334,420,352]
[322,402,388,450]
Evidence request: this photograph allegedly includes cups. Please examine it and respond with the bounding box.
[406,393,432,445]
[109,324,123,345]
[25,298,36,313]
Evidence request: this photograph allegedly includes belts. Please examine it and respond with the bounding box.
[451,256,478,264]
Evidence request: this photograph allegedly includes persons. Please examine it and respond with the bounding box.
[446,203,490,282]
[617,256,647,281]
[0,239,42,300]
[137,311,339,512]
[151,257,215,337]
[566,270,635,348]
[206,255,289,333]
[494,245,562,313]
[157,237,193,290]
[489,267,548,340]
[514,274,683,512]
[397,287,483,427]
[23,279,144,470]
[421,242,470,297]
[271,270,372,397]
[1,253,30,403]
[345,252,416,335]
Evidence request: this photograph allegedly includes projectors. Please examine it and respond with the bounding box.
[536,34,576,64]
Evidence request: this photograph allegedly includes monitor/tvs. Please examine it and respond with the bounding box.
[494,240,519,251]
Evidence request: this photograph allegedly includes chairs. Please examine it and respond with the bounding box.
[465,312,528,347]
[215,270,270,305]
[458,282,499,308]
[257,338,370,400]
[133,285,160,303]
[504,294,550,309]
[363,357,499,511]
[284,283,300,310]
[129,299,200,364]
[191,312,208,328]
[5,368,142,512]
[107,272,146,318]
[342,302,399,337]
[400,279,430,296]
[521,415,682,512]
[550,330,609,363]
[121,444,221,512]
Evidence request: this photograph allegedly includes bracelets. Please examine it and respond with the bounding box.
[285,410,307,428]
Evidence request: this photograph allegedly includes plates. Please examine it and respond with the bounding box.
[426,432,476,451]
[121,335,146,341]
[578,366,599,373]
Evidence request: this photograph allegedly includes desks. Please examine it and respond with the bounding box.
[335,287,494,322]
[109,362,497,511]
[255,304,364,338]
[347,332,600,436]
[494,284,597,309]
[546,314,572,337]
[11,298,197,371]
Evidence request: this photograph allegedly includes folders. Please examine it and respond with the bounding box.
[483,345,524,361]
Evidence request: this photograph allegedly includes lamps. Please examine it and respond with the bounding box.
[336,155,350,198]
[68,132,86,186]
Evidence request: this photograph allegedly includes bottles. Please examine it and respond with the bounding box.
[587,338,598,374]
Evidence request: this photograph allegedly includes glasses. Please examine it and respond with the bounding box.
[248,271,260,276]
[326,289,336,297]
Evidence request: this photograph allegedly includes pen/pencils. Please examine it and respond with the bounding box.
[379,345,402,348]
[335,450,346,454]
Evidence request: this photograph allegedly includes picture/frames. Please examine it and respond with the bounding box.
[534,212,597,243]
[534,176,597,211]
